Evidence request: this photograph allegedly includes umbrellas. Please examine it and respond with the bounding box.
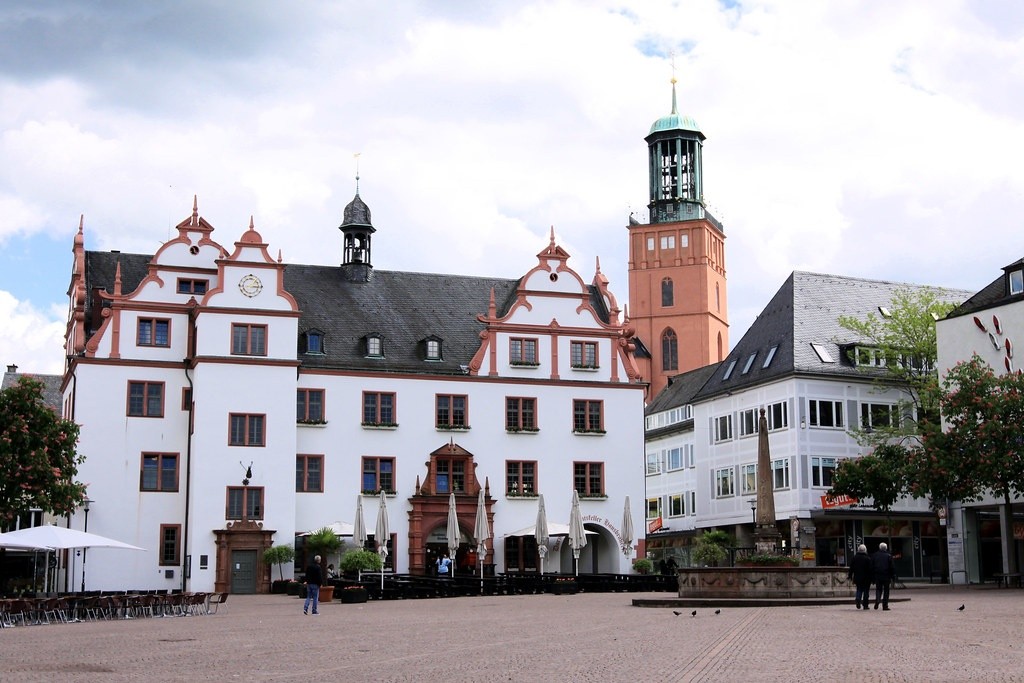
[534,493,549,580]
[620,495,634,573]
[474,488,490,592]
[445,492,461,577]
[352,494,368,581]
[374,490,391,589]
[569,490,587,575]
[0,521,148,598]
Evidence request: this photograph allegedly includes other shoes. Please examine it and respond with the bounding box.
[883,607,891,611]
[856,603,861,609]
[863,606,871,610]
[874,605,878,609]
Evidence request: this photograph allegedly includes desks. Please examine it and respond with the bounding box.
[996,573,1021,589]
[360,572,679,599]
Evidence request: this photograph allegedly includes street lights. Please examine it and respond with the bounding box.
[746,499,757,529]
[80,500,96,592]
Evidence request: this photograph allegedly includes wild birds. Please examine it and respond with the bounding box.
[673,609,721,617]
[957,604,965,611]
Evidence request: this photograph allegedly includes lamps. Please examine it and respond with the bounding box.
[239,460,253,479]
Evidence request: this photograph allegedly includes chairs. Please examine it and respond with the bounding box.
[0,590,230,630]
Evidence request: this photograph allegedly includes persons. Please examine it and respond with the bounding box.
[659,555,679,575]
[435,553,450,586]
[872,542,896,610]
[845,544,872,610]
[326,564,336,585]
[302,555,322,615]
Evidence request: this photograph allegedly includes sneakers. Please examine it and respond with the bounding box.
[304,610,308,615]
[312,611,320,615]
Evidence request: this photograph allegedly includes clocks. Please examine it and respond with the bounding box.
[239,274,263,298]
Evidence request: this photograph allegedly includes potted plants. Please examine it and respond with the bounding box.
[633,558,654,592]
[265,527,383,605]
